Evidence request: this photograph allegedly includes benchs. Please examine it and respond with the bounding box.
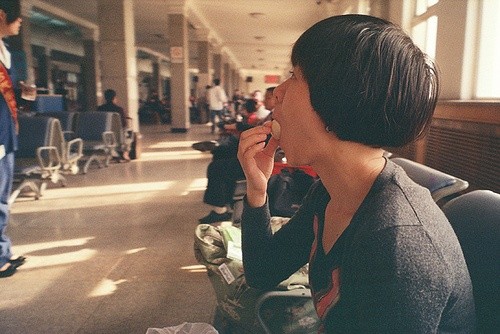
[5,110,136,207]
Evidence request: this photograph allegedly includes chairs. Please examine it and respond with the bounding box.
[441,187,500,334]
[254,155,471,334]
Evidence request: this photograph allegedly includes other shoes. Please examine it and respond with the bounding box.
[192,141,216,152]
[0,255,26,276]
[199,210,231,223]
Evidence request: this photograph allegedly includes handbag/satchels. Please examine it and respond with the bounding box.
[266,165,319,219]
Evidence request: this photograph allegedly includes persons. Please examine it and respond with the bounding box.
[0,0,26,278]
[198,141,247,224]
[206,78,228,134]
[96,89,130,161]
[222,87,275,140]
[189,85,212,124]
[237,14,476,334]
[139,88,171,122]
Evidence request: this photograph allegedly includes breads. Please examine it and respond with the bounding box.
[271,119,281,140]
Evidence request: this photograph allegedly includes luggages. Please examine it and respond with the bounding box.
[125,117,142,159]
